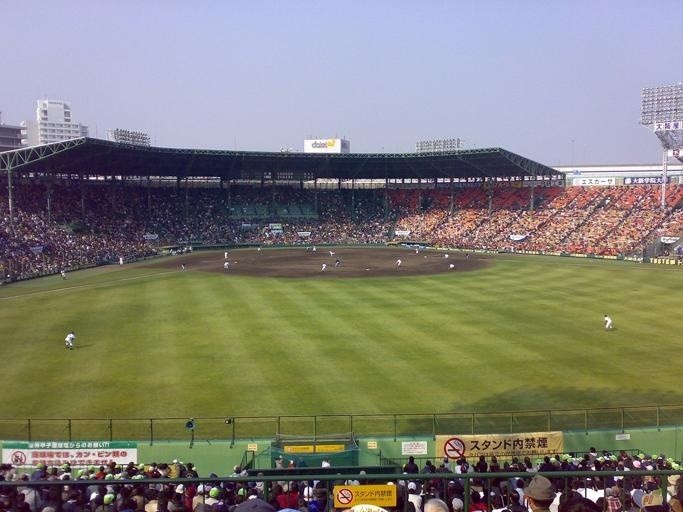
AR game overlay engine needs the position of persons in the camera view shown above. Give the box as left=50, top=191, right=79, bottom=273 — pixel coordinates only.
left=424, top=185, right=682, bottom=264
left=334, top=259, right=341, bottom=268
left=394, top=258, right=401, bottom=272
left=224, top=251, right=228, bottom=262
left=2, top=447, right=683, bottom=512
left=602, top=314, right=612, bottom=330
left=0, top=170, right=193, bottom=284
left=328, top=250, right=335, bottom=256
left=63, top=330, right=75, bottom=350
left=223, top=261, right=230, bottom=272
left=192, top=185, right=423, bottom=255
left=447, top=264, right=455, bottom=272
left=320, top=263, right=327, bottom=272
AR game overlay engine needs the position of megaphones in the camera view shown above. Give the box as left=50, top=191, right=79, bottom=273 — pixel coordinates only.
left=186, top=421, right=195, bottom=430
